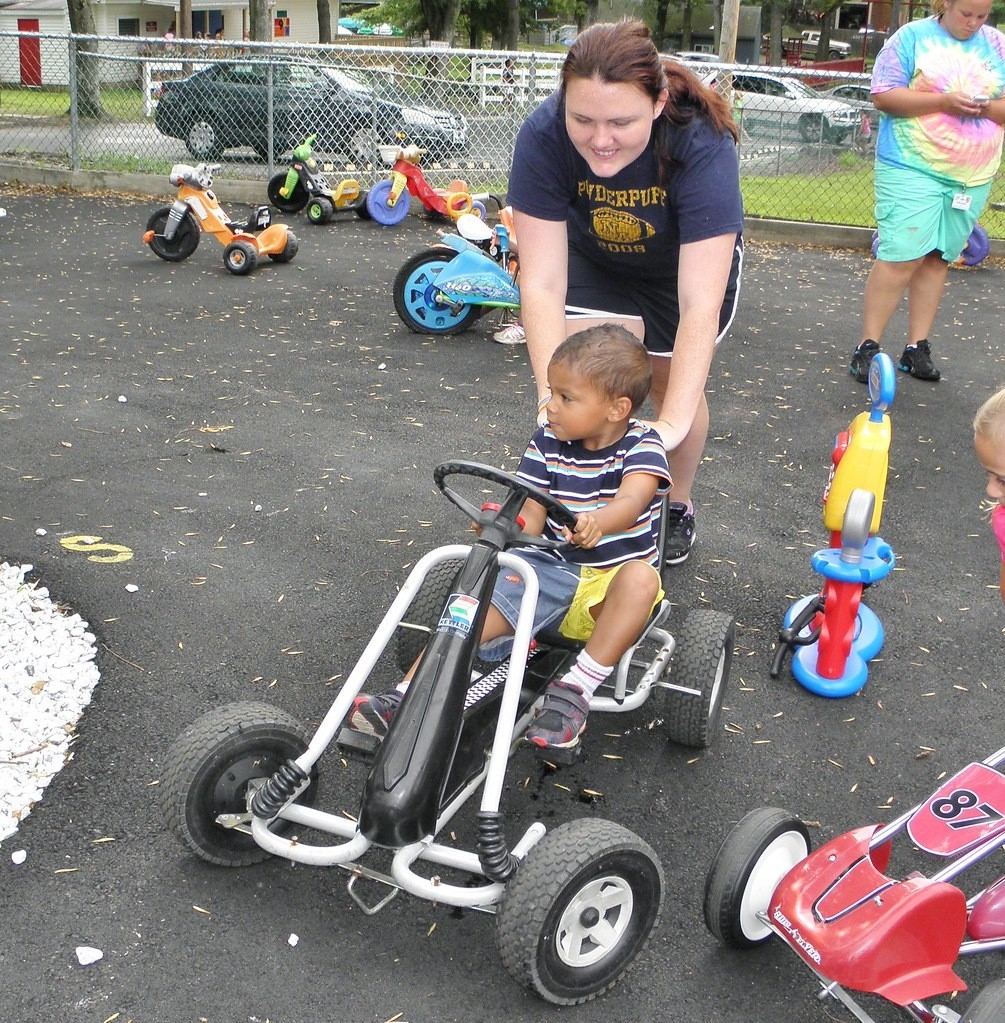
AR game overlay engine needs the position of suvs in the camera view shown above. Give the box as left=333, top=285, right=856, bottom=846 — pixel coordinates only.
left=701, top=70, right=864, bottom=146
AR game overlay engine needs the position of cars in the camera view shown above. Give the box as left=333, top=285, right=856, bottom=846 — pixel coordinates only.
left=154, top=52, right=472, bottom=165
left=550, top=25, right=579, bottom=46
left=659, top=49, right=881, bottom=126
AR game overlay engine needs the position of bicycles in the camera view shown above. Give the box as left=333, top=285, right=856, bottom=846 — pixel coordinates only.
left=393, top=191, right=524, bottom=335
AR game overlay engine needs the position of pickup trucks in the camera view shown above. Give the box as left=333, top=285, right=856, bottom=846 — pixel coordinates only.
left=762, top=27, right=853, bottom=60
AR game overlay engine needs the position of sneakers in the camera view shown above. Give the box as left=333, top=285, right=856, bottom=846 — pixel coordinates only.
left=525, top=680, right=590, bottom=747
left=850, top=339, right=881, bottom=383
left=898, top=339, right=940, bottom=382
left=349, top=687, right=406, bottom=738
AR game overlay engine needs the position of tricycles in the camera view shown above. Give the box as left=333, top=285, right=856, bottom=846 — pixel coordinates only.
left=367, top=143, right=487, bottom=225
left=142, top=161, right=298, bottom=276
left=867, top=217, right=991, bottom=268
left=268, top=133, right=375, bottom=224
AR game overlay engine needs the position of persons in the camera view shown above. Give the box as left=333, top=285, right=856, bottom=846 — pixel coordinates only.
left=502, top=57, right=519, bottom=116
left=848, top=1, right=1004, bottom=385
left=970, top=385, right=1005, bottom=611
left=342, top=323, right=679, bottom=752
left=164, top=24, right=255, bottom=60
left=503, top=17, right=747, bottom=571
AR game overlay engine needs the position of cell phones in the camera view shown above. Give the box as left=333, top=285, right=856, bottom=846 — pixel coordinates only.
left=971, top=95, right=989, bottom=102
